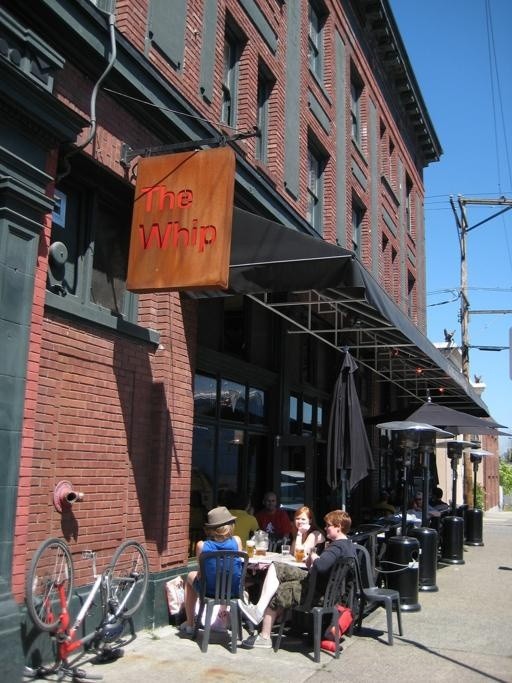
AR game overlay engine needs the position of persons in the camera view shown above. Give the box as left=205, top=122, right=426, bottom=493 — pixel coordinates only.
left=236, top=509, right=356, bottom=648
left=430, top=488, right=449, bottom=511
left=412, top=491, right=441, bottom=518
left=178, top=505, right=242, bottom=638
left=374, top=494, right=397, bottom=514
left=290, top=506, right=328, bottom=562
left=254, top=490, right=292, bottom=537
left=228, top=495, right=261, bottom=546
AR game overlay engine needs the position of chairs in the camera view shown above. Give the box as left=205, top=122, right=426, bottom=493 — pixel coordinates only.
left=274, top=556, right=354, bottom=663
left=193, top=551, right=249, bottom=653
left=266, top=502, right=471, bottom=558
left=350, top=544, right=403, bottom=646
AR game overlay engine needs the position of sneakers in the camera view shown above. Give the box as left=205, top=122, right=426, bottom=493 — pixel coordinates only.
left=238, top=599, right=263, bottom=626
left=180, top=624, right=195, bottom=638
left=242, top=633, right=272, bottom=649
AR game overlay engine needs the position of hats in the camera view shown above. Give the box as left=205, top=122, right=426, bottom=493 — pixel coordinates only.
left=204, top=506, right=238, bottom=526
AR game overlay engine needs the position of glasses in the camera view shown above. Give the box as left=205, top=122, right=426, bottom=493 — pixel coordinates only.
left=326, top=523, right=334, bottom=527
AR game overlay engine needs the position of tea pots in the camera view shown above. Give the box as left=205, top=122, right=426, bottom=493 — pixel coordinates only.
left=254, top=530, right=268, bottom=557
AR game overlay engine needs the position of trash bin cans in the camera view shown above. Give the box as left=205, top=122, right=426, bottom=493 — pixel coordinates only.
left=439, top=516, right=465, bottom=564
left=465, top=509, right=484, bottom=546
left=385, top=527, right=438, bottom=612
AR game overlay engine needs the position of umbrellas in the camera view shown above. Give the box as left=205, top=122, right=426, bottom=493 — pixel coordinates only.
left=328, top=345, right=378, bottom=510
left=438, top=425, right=512, bottom=438
left=361, top=400, right=509, bottom=429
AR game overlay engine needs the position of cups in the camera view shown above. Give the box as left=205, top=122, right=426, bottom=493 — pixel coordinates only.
left=282, top=545, right=290, bottom=559
left=294, top=548, right=305, bottom=563
left=245, top=541, right=254, bottom=558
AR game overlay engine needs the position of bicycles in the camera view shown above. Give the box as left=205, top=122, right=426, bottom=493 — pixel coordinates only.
left=22, top=537, right=150, bottom=680
